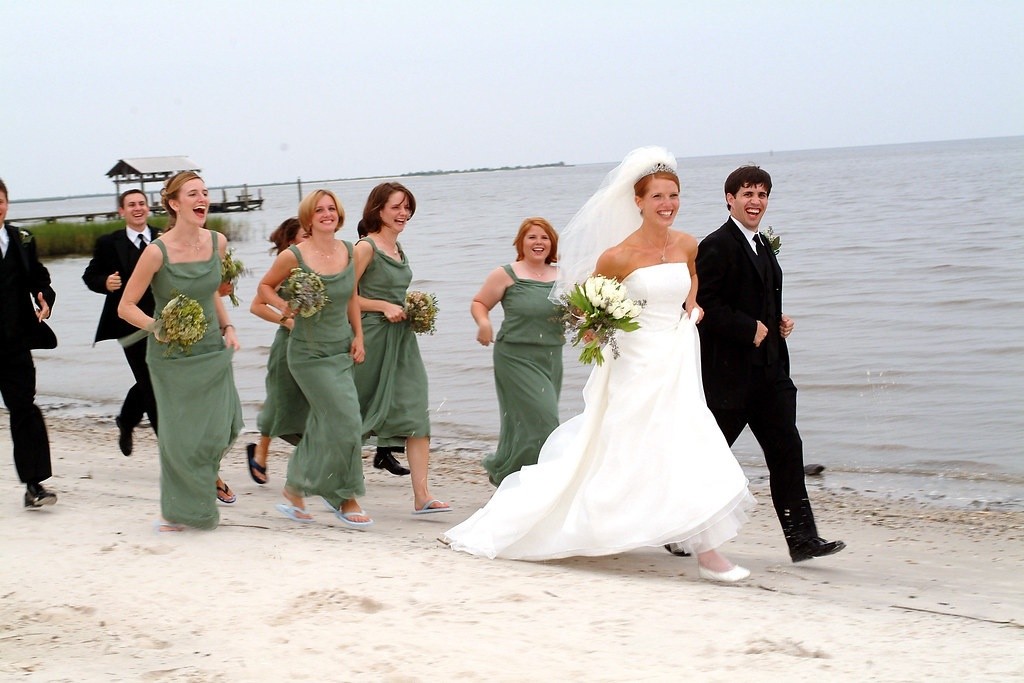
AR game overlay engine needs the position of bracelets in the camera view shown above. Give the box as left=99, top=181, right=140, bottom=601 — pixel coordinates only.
left=224, top=325, right=234, bottom=330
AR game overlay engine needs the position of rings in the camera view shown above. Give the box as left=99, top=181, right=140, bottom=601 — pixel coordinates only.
left=788, top=331, right=790, bottom=334
left=584, top=334, right=587, bottom=338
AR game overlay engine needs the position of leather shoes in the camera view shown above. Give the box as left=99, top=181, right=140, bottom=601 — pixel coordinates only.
left=791, top=536, right=847, bottom=562
left=664, top=541, right=692, bottom=557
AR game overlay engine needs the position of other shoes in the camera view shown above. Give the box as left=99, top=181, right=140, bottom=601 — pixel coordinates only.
left=697, top=561, right=752, bottom=584
left=373, top=451, right=412, bottom=477
left=115, top=415, right=133, bottom=457
left=23, top=484, right=58, bottom=511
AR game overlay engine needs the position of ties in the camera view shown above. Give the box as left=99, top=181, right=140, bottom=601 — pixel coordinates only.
left=137, top=234, right=147, bottom=249
left=750, top=233, right=771, bottom=284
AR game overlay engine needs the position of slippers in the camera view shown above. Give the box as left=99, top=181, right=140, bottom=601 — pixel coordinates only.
left=334, top=508, right=374, bottom=527
left=246, top=444, right=267, bottom=484
left=272, top=498, right=315, bottom=524
left=157, top=515, right=182, bottom=532
left=215, top=478, right=236, bottom=504
left=412, top=499, right=454, bottom=515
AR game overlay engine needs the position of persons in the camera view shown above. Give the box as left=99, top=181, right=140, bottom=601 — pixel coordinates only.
left=472, top=216, right=579, bottom=489
left=246, top=182, right=452, bottom=528
left=0, top=178, right=57, bottom=507
left=442, top=145, right=758, bottom=582
left=116, top=170, right=244, bottom=531
left=661, top=165, right=845, bottom=564
left=82, top=189, right=165, bottom=455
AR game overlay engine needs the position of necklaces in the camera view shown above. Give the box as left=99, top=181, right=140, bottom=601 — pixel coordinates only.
left=183, top=236, right=200, bottom=250
left=640, top=227, right=669, bottom=261
left=523, top=259, right=549, bottom=278
left=311, top=244, right=338, bottom=259
left=395, top=251, right=396, bottom=254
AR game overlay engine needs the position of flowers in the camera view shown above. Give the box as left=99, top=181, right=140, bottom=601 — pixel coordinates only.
left=279, top=266, right=333, bottom=324
left=19, top=230, right=35, bottom=247
left=157, top=289, right=211, bottom=357
left=382, top=290, right=439, bottom=336
left=763, top=226, right=781, bottom=255
left=561, top=273, right=643, bottom=367
left=222, top=247, right=244, bottom=306
